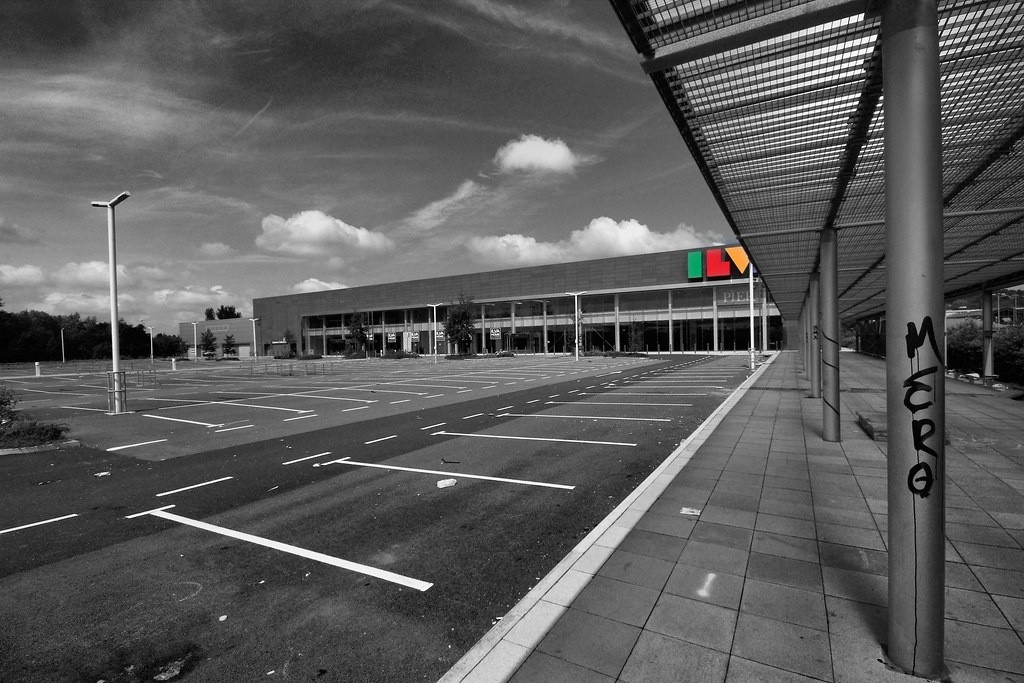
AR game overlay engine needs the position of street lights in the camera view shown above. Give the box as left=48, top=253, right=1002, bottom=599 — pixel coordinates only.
left=148, top=326, right=155, bottom=363
left=91, top=191, right=132, bottom=414
left=191, top=322, right=200, bottom=362
left=248, top=317, right=259, bottom=365
left=564, top=291, right=588, bottom=361
left=427, top=303, right=442, bottom=363
